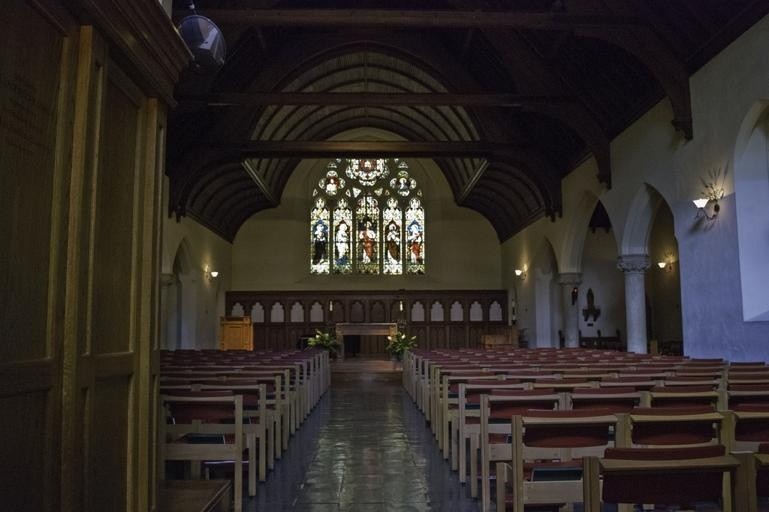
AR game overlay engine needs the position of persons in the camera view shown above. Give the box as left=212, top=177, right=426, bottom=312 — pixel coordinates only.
left=313, top=220, right=422, bottom=265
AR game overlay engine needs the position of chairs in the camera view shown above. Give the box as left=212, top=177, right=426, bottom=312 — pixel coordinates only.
left=394, top=345, right=769, bottom=511
left=160, top=349, right=330, bottom=507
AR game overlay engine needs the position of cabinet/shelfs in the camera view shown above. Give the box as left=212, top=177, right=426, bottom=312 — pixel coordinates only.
left=1, top=2, right=206, bottom=512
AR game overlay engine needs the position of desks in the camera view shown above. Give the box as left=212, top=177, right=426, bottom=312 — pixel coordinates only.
left=335, top=323, right=398, bottom=356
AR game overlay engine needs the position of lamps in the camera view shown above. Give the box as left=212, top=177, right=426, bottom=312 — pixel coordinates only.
left=693, top=194, right=722, bottom=222
left=514, top=269, right=528, bottom=281
